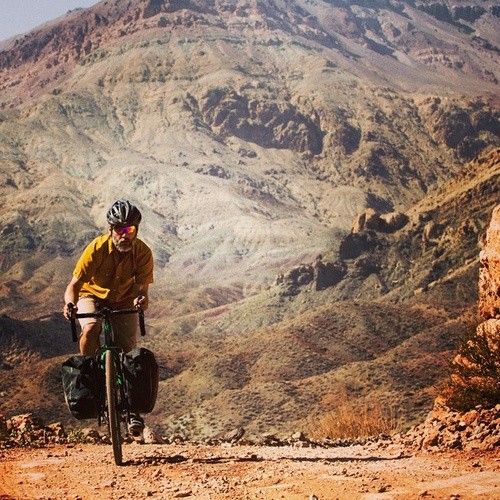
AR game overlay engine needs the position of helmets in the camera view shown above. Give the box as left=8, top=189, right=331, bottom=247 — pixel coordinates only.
left=106, top=198, right=142, bottom=225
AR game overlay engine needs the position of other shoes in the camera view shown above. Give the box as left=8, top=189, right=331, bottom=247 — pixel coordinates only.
left=128, top=410, right=145, bottom=437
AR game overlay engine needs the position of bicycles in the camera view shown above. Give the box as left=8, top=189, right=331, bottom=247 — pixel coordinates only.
left=64, top=296, right=146, bottom=465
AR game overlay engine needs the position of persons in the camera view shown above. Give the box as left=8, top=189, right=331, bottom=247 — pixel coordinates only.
left=62, top=199, right=155, bottom=438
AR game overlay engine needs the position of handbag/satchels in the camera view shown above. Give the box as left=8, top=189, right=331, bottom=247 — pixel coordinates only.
left=61, top=355, right=104, bottom=420
left=122, top=347, right=158, bottom=413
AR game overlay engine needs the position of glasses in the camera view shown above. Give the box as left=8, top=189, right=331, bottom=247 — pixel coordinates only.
left=111, top=225, right=136, bottom=235
